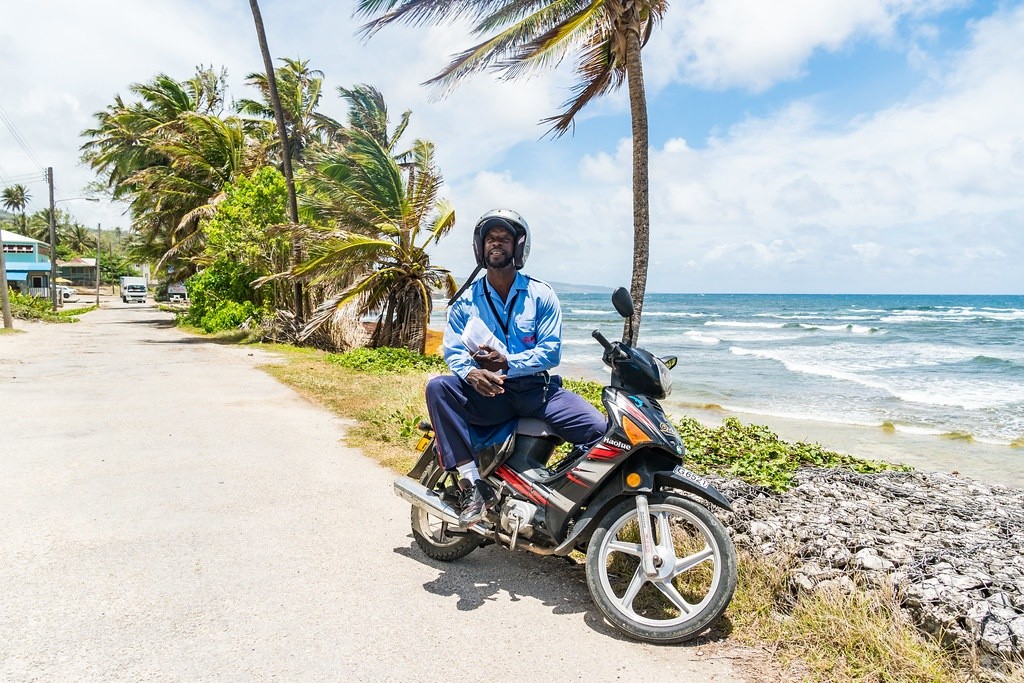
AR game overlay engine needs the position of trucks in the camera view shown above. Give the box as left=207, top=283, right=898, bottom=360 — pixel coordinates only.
left=121, top=278, right=147, bottom=303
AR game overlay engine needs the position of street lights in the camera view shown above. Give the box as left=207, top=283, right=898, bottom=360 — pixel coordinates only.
left=49, top=198, right=100, bottom=312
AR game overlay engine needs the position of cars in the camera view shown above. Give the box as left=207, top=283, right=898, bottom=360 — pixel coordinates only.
left=56, top=285, right=77, bottom=299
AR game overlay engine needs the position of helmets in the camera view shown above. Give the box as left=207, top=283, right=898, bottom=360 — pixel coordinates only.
left=472, top=207, right=531, bottom=270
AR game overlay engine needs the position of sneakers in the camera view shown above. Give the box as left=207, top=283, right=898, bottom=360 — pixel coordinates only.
left=454, top=478, right=502, bottom=528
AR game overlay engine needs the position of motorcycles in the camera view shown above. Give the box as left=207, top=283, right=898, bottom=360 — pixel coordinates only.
left=392, top=281, right=739, bottom=646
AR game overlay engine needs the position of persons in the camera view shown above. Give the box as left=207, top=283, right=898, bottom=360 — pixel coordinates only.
left=424, top=207, right=608, bottom=530
